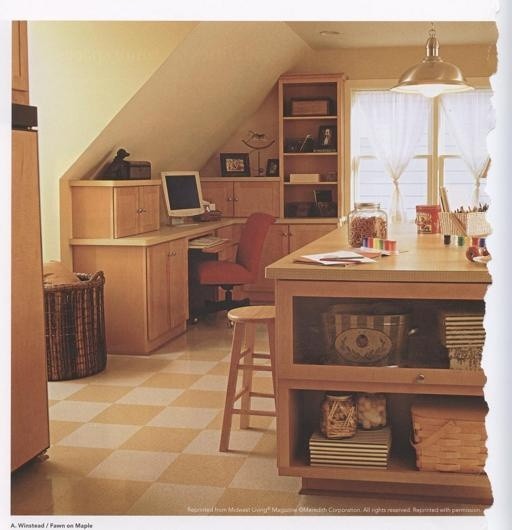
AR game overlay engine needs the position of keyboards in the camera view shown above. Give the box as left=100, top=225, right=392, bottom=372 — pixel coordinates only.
left=190, top=235, right=224, bottom=249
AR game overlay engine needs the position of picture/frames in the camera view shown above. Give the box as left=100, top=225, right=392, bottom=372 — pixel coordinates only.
left=219, top=152, right=251, bottom=176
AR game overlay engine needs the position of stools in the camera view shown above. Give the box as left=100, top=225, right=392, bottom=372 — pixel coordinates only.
left=218, top=307, right=275, bottom=453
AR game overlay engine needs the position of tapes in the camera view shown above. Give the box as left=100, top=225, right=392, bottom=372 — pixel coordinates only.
left=465, top=247, right=480, bottom=263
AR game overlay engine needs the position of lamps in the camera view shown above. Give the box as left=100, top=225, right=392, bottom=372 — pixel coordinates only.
left=386, top=22, right=474, bottom=97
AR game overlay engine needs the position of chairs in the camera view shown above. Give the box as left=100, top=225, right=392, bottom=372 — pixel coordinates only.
left=195, top=213, right=277, bottom=329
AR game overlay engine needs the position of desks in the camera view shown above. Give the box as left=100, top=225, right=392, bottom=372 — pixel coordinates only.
left=69, top=217, right=233, bottom=354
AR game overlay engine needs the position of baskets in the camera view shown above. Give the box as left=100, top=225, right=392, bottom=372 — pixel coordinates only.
left=43, top=270, right=108, bottom=381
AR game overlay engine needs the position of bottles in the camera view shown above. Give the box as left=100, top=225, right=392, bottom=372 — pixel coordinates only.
left=347, top=202, right=390, bottom=249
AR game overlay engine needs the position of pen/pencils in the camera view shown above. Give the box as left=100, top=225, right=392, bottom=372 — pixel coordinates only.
left=439, top=187, right=489, bottom=213
left=320, top=257, right=363, bottom=263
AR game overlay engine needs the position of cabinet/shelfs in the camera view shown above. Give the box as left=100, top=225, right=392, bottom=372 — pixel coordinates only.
left=264, top=222, right=493, bottom=503
left=277, top=71, right=348, bottom=225
left=235, top=223, right=340, bottom=304
left=68, top=179, right=279, bottom=238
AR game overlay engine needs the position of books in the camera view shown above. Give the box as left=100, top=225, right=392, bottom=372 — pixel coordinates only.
left=301, top=250, right=377, bottom=265
left=293, top=248, right=382, bottom=268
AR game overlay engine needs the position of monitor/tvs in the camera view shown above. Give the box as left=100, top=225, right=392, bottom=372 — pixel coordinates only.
left=160, top=171, right=205, bottom=226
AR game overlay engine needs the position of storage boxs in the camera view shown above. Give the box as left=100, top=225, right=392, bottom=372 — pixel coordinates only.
left=307, top=419, right=392, bottom=469
left=439, top=307, right=485, bottom=371
